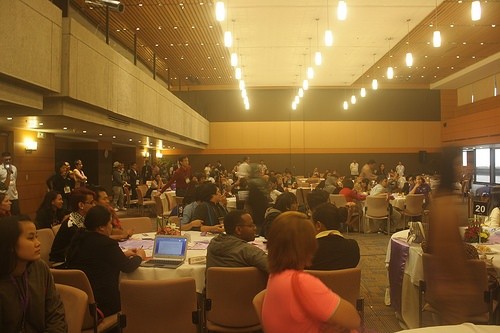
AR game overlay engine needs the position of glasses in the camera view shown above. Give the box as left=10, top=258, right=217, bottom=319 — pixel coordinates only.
left=235, top=224, right=257, bottom=228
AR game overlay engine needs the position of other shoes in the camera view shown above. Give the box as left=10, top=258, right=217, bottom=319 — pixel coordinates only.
left=119, top=207, right=127, bottom=210
left=114, top=207, right=119, bottom=212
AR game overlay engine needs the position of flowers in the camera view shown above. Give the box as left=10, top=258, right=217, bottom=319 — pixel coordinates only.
left=465, top=227, right=490, bottom=244
left=157, top=217, right=183, bottom=239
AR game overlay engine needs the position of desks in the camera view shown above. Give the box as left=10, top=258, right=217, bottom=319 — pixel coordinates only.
left=389, top=237, right=500, bottom=319
left=110, top=232, right=268, bottom=291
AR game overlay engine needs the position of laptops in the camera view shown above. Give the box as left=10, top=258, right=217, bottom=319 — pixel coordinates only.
left=139, top=235, right=188, bottom=270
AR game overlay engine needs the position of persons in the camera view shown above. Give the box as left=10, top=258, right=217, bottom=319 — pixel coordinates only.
left=305, top=203, right=364, bottom=270
left=262, top=193, right=298, bottom=238
left=488, top=206, right=500, bottom=228
left=202, top=210, right=270, bottom=319
left=253, top=209, right=361, bottom=333
left=111, top=154, right=302, bottom=212
left=91, top=185, right=135, bottom=242
left=0, top=191, right=11, bottom=216
left=46, top=159, right=87, bottom=220
left=37, top=190, right=64, bottom=230
left=0, top=214, right=67, bottom=333
left=48, top=189, right=95, bottom=270
left=181, top=181, right=226, bottom=233
left=308, top=158, right=431, bottom=235
left=65, top=205, right=146, bottom=333
left=0, top=150, right=19, bottom=216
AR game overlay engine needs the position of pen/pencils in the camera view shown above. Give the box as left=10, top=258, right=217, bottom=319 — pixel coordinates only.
left=140, top=246, right=144, bottom=250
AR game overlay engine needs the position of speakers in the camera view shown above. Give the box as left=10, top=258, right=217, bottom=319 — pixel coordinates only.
left=419, top=150, right=427, bottom=163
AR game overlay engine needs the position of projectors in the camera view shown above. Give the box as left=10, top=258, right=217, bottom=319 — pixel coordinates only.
left=85, top=0, right=125, bottom=14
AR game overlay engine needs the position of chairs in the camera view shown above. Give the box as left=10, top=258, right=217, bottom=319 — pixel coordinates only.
left=125, top=186, right=175, bottom=218
left=50, top=266, right=362, bottom=333
left=421, top=253, right=495, bottom=326
left=298, top=188, right=429, bottom=235
